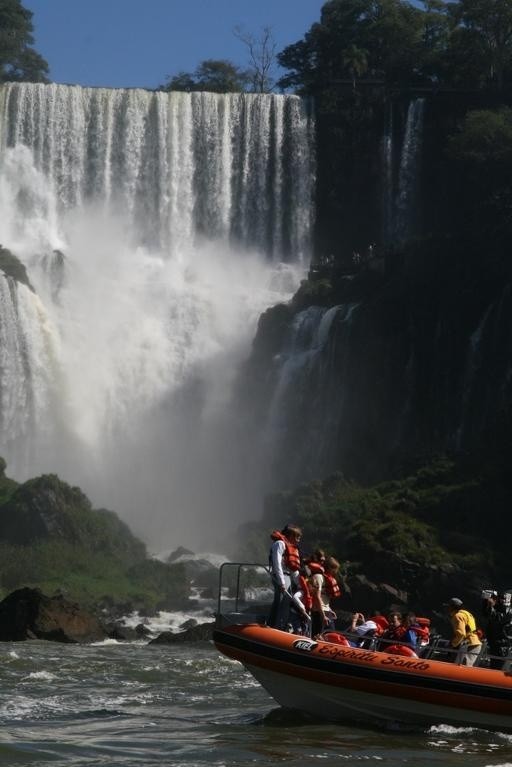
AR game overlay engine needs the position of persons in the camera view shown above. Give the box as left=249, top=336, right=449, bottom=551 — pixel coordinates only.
left=293, top=548, right=512, bottom=670
left=269, top=525, right=303, bottom=632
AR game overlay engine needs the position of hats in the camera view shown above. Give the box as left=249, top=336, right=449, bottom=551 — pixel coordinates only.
left=442, top=598, right=463, bottom=608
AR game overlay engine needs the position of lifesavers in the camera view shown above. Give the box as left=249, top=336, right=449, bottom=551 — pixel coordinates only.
left=383, top=645, right=418, bottom=658
left=322, top=633, right=350, bottom=647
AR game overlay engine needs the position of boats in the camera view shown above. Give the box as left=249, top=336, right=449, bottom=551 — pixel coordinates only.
left=212, top=562, right=512, bottom=735
